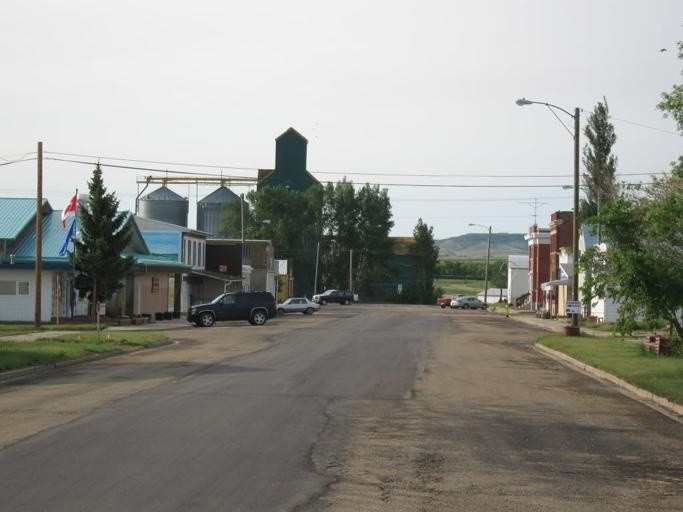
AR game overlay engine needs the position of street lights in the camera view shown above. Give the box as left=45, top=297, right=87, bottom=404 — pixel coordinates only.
left=515, top=97, right=582, bottom=335
left=559, top=182, right=602, bottom=247
left=468, top=223, right=492, bottom=306
left=240, top=218, right=271, bottom=245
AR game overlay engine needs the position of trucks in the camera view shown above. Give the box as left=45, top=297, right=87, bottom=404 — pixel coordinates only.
left=435, top=292, right=465, bottom=308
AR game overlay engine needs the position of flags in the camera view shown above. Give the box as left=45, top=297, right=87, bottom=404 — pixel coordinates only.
left=58, top=218, right=76, bottom=257
left=60, top=193, right=77, bottom=231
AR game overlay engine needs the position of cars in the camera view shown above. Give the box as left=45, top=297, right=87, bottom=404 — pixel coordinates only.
left=450, top=296, right=489, bottom=310
left=277, top=298, right=320, bottom=314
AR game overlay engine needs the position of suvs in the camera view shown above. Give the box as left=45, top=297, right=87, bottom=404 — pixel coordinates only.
left=187, top=290, right=277, bottom=327
left=312, top=289, right=353, bottom=305
